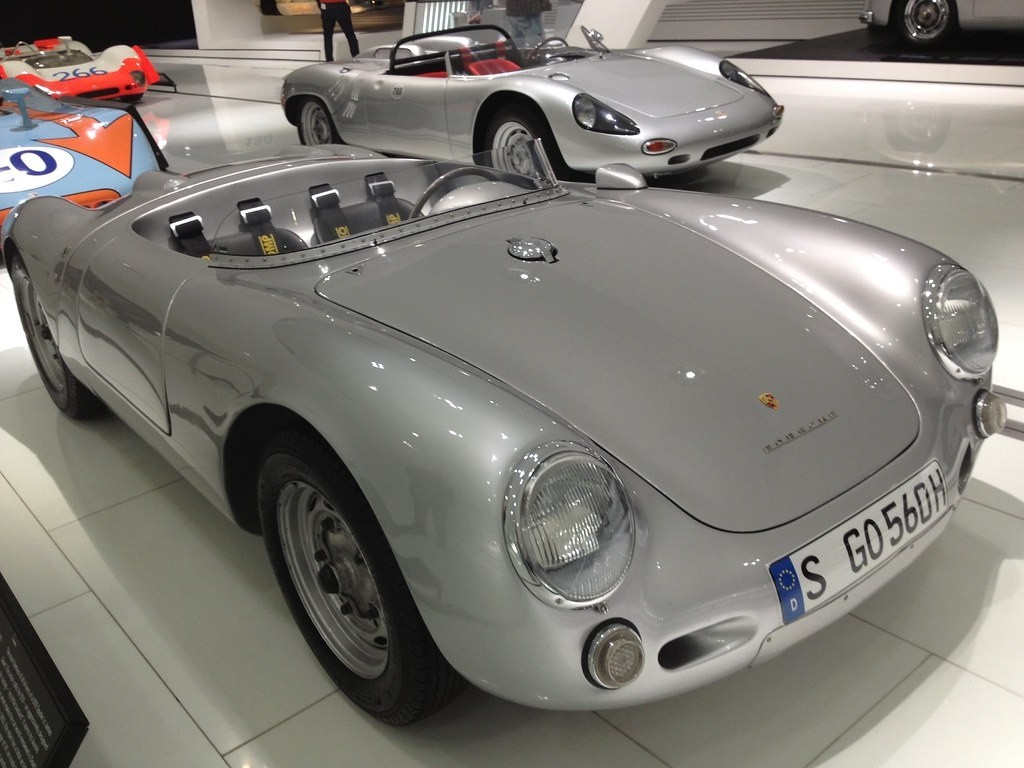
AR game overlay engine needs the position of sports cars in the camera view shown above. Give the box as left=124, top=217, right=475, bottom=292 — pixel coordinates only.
left=1, top=78, right=170, bottom=245
left=0, top=35, right=160, bottom=104
left=280, top=24, right=786, bottom=183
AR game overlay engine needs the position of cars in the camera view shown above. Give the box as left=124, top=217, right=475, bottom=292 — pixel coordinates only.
left=1, top=141, right=1011, bottom=725
left=857, top=0, right=1024, bottom=53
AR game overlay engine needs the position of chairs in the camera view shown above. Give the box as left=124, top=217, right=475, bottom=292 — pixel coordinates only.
left=470, top=56, right=521, bottom=74
left=183, top=227, right=310, bottom=258
left=314, top=199, right=423, bottom=245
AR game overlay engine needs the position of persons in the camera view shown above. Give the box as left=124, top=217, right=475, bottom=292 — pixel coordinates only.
left=506, top=0, right=552, bottom=48
left=317, top=0, right=360, bottom=62
